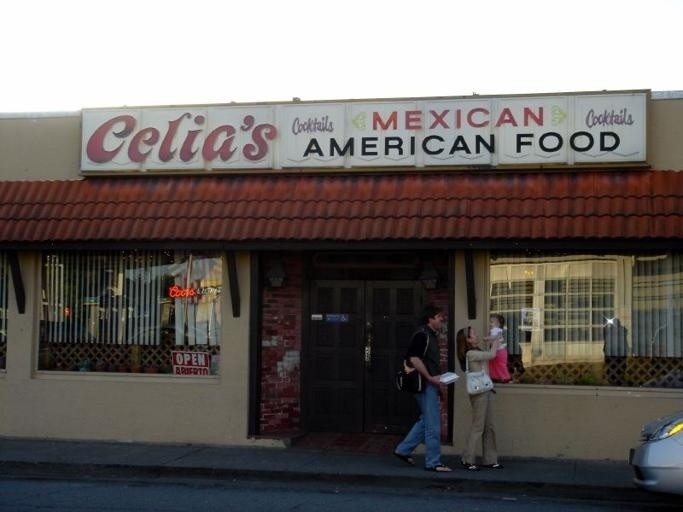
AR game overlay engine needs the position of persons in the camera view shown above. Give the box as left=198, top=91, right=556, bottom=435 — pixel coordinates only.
left=394, top=306, right=454, bottom=472
left=482, top=313, right=510, bottom=383
left=457, top=326, right=503, bottom=470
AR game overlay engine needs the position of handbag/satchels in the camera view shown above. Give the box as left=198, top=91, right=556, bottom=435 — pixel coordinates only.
left=396, top=360, right=425, bottom=394
left=465, top=351, right=494, bottom=395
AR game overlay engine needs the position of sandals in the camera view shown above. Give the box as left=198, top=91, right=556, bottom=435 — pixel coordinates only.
left=393, top=449, right=415, bottom=465
left=424, top=464, right=453, bottom=472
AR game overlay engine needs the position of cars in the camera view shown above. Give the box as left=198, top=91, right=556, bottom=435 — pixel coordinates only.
left=629, top=411, right=683, bottom=496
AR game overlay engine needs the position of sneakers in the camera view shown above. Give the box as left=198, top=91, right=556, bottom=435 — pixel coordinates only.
left=464, top=463, right=480, bottom=471
left=483, top=463, right=503, bottom=469
